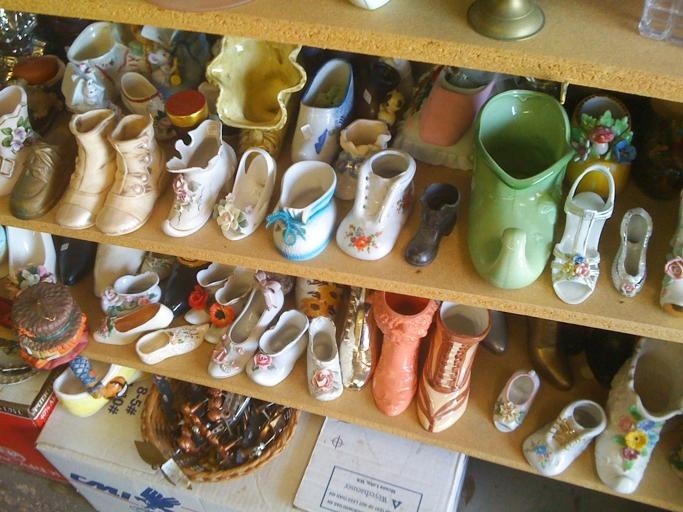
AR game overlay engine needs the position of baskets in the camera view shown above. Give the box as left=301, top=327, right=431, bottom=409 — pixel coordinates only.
left=141, top=375, right=301, bottom=483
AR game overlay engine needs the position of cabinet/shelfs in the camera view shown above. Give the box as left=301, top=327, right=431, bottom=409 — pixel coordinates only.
left=0, top=2, right=683, bottom=512
